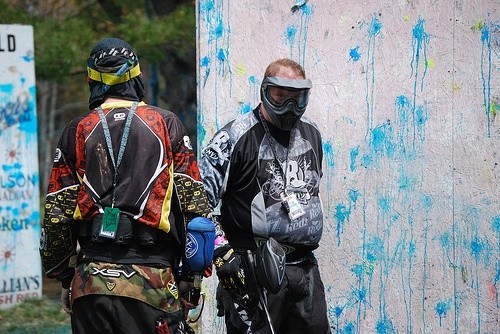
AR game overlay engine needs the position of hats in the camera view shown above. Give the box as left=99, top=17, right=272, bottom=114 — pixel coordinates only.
left=86, top=37, right=145, bottom=110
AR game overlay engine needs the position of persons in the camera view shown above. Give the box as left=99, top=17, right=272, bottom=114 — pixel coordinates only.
left=199, top=59, right=332, bottom=334
left=39, top=38, right=211, bottom=334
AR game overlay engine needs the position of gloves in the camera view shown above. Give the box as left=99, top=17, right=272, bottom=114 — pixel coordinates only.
left=213, top=244, right=247, bottom=292
